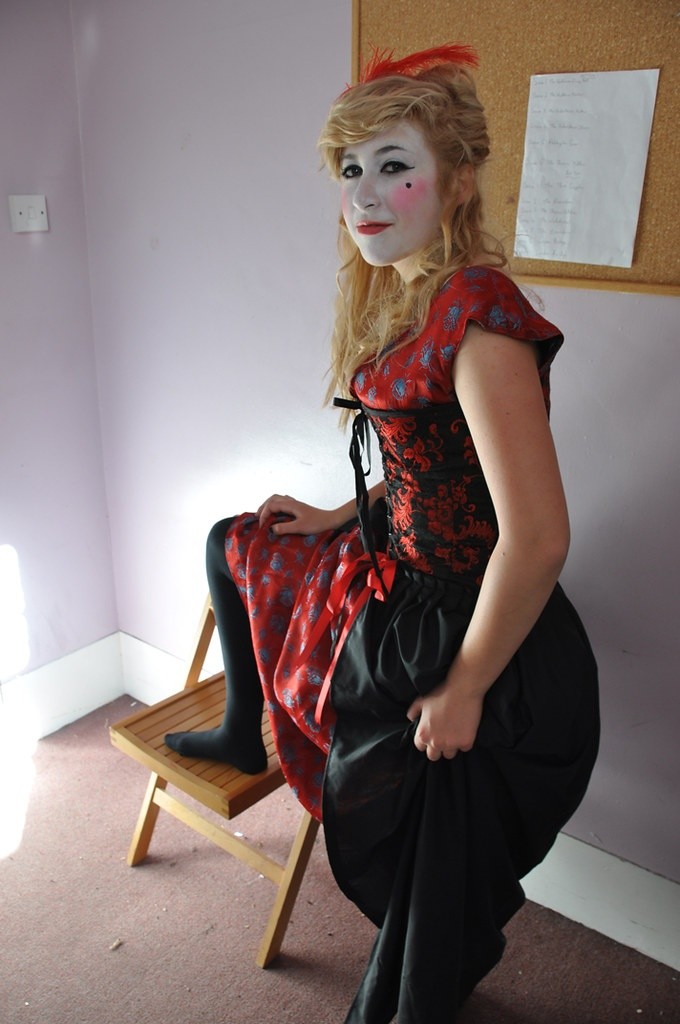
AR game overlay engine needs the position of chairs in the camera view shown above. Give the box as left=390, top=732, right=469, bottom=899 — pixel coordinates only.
left=104, top=585, right=322, bottom=973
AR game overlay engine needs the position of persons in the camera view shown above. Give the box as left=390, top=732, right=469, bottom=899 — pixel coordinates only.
left=164, top=42, right=601, bottom=1024
